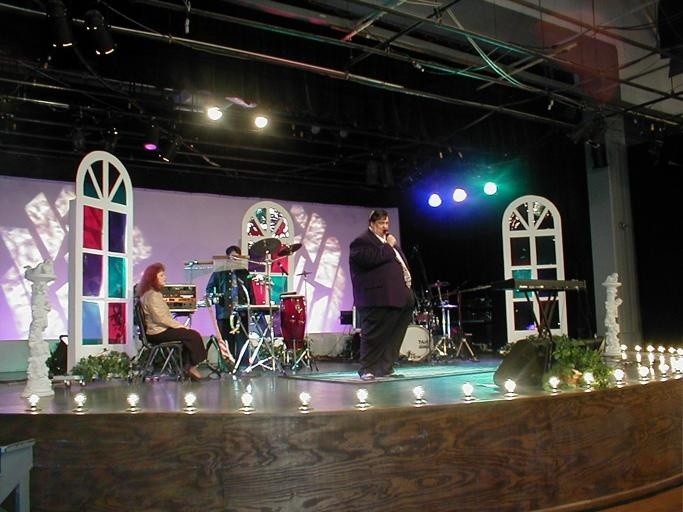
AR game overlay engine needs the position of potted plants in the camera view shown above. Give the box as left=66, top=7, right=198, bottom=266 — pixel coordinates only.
left=530, top=332, right=612, bottom=393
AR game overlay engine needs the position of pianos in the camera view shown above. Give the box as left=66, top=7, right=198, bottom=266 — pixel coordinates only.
left=492, top=278, right=586, bottom=290
left=232, top=303, right=280, bottom=313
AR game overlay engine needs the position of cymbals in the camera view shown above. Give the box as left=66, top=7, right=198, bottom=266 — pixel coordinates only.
left=251, top=238, right=281, bottom=255
left=278, top=243, right=303, bottom=256
left=297, top=272, right=313, bottom=276
left=415, top=314, right=436, bottom=323
left=438, top=305, right=459, bottom=308
left=213, top=263, right=247, bottom=272
left=213, top=255, right=246, bottom=259
left=428, top=282, right=451, bottom=287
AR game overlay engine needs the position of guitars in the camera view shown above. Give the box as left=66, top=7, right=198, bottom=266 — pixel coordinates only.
left=203, top=295, right=237, bottom=372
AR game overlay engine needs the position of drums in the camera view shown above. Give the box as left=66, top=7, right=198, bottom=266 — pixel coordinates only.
left=280, top=295, right=307, bottom=349
left=450, top=327, right=461, bottom=339
left=399, top=325, right=434, bottom=362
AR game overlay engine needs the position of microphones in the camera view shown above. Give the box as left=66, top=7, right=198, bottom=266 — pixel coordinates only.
left=383, top=229, right=400, bottom=249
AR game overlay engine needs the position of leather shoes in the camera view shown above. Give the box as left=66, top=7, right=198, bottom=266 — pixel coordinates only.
left=361, top=372, right=375, bottom=380
left=183, top=370, right=211, bottom=382
left=388, top=371, right=404, bottom=378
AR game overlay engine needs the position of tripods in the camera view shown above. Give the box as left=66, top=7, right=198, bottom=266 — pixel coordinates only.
left=429, top=286, right=457, bottom=357
left=228, top=263, right=286, bottom=375
left=291, top=278, right=320, bottom=373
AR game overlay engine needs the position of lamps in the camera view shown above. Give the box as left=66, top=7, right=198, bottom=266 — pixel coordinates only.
left=636, top=352, right=641, bottom=360
left=126, top=393, right=143, bottom=411
left=677, top=348, right=683, bottom=354
left=648, top=353, right=655, bottom=362
left=678, top=357, right=683, bottom=362
left=145, top=124, right=160, bottom=149
left=36, top=31, right=77, bottom=70
left=660, top=354, right=666, bottom=363
left=659, top=363, right=669, bottom=378
left=84, top=19, right=118, bottom=55
left=297, top=392, right=315, bottom=414
left=635, top=345, right=641, bottom=352
left=658, top=346, right=666, bottom=353
left=410, top=386, right=427, bottom=407
left=621, top=343, right=627, bottom=352
left=353, top=387, right=371, bottom=410
left=28, top=393, right=41, bottom=412
left=668, top=347, right=675, bottom=353
left=457, top=381, right=476, bottom=401
left=670, top=355, right=676, bottom=361
left=502, top=379, right=521, bottom=399
left=638, top=366, right=650, bottom=382
left=237, top=391, right=256, bottom=413
left=548, top=377, right=562, bottom=395
left=621, top=352, right=628, bottom=360
left=613, top=369, right=627, bottom=385
left=180, top=392, right=201, bottom=414
left=672, top=363, right=683, bottom=374
left=156, top=138, right=177, bottom=163
left=647, top=345, right=655, bottom=353
left=581, top=373, right=597, bottom=392
left=72, top=393, right=89, bottom=412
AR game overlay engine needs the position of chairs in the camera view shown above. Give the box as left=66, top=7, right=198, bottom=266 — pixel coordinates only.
left=130, top=302, right=182, bottom=381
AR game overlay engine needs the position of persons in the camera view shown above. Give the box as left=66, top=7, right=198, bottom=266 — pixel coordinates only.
left=348, top=209, right=415, bottom=380
left=138, top=263, right=206, bottom=382
left=206, top=246, right=256, bottom=371
